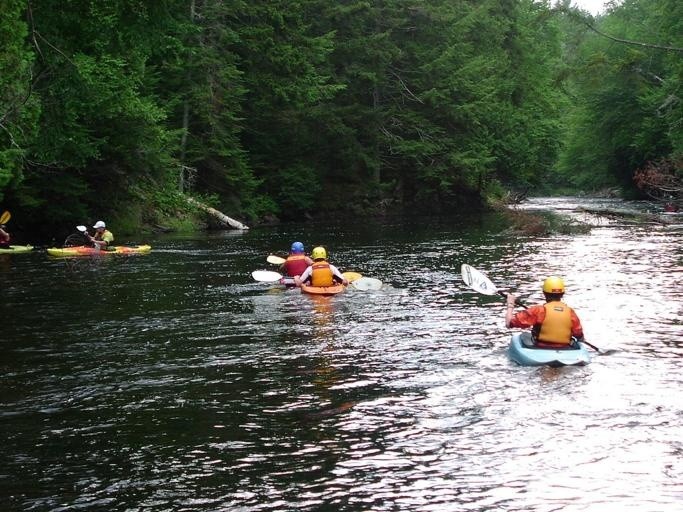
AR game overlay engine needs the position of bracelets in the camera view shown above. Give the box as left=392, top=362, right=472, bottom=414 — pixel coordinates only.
left=507, top=302, right=513, bottom=308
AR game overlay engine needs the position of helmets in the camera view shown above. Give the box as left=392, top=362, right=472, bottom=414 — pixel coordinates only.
left=543, top=276, right=564, bottom=294
left=291, top=242, right=304, bottom=255
left=312, top=247, right=326, bottom=260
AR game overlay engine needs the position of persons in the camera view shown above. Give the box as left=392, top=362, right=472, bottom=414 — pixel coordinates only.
left=294, top=247, right=344, bottom=286
left=83, top=221, right=114, bottom=250
left=504, top=276, right=585, bottom=351
left=277, top=241, right=315, bottom=278
left=0, top=225, right=10, bottom=246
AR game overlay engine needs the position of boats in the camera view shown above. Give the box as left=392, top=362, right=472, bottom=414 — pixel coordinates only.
left=280, top=275, right=310, bottom=289
left=297, top=270, right=362, bottom=296
left=0, top=245, right=39, bottom=255
left=46, top=243, right=155, bottom=256
left=504, top=323, right=591, bottom=370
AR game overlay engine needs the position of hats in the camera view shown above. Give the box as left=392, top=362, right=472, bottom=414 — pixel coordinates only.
left=93, top=221, right=106, bottom=228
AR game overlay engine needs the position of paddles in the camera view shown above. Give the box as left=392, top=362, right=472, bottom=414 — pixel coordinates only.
left=460, top=264, right=605, bottom=355
left=76, top=225, right=101, bottom=254
left=0, top=210, right=11, bottom=224
left=252, top=255, right=382, bottom=291
